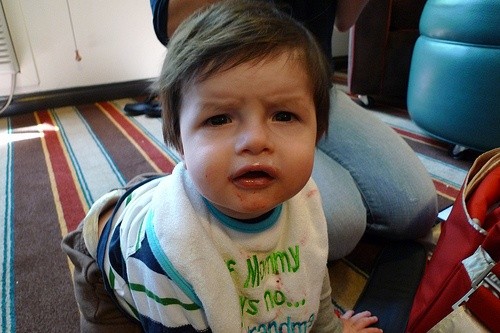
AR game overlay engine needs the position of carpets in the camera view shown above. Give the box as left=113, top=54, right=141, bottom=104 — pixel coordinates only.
left=1, top=67, right=480, bottom=333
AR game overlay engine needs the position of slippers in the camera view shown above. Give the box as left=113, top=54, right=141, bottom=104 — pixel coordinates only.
left=124, top=93, right=163, bottom=116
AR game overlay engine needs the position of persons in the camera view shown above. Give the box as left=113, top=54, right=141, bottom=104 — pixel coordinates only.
left=149, top=0, right=438, bottom=262
left=61, top=0, right=383, bottom=333
